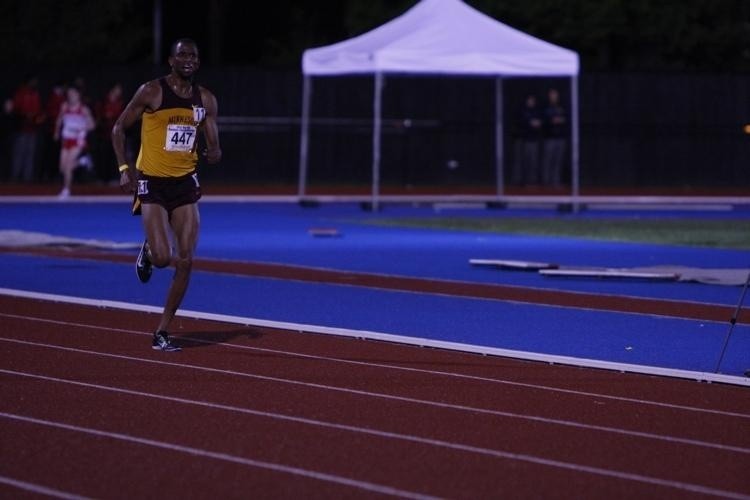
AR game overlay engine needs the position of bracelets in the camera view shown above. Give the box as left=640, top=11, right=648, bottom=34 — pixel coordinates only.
left=118, top=164, right=129, bottom=173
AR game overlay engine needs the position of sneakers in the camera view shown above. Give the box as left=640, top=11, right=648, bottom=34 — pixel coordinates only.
left=135, top=236, right=158, bottom=285
left=151, top=330, right=183, bottom=353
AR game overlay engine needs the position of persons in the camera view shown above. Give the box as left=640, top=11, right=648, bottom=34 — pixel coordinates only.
left=540, top=88, right=567, bottom=186
left=93, top=82, right=125, bottom=182
left=510, top=93, right=545, bottom=186
left=2, top=71, right=96, bottom=199
left=110, top=38, right=222, bottom=352
